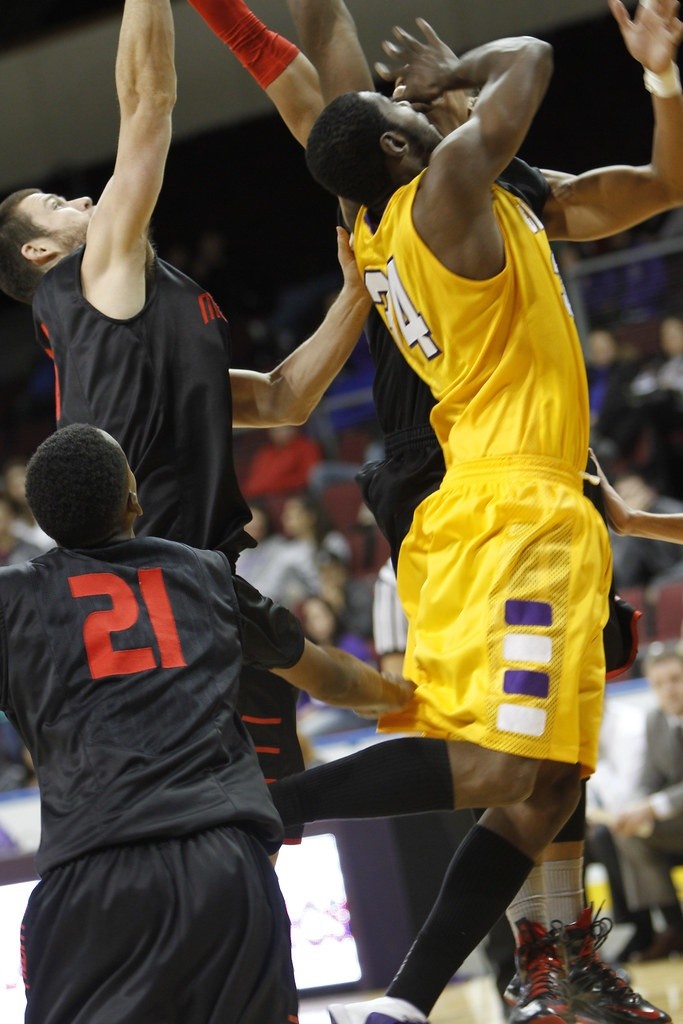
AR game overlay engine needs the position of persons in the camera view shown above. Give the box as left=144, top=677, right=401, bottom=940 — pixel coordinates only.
left=0, top=0, right=683, bottom=1024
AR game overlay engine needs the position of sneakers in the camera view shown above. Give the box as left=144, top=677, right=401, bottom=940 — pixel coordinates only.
left=503, top=917, right=575, bottom=1024
left=548, top=901, right=673, bottom=1024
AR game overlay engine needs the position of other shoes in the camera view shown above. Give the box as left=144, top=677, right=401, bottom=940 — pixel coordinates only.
left=640, top=927, right=677, bottom=960
left=327, top=996, right=428, bottom=1024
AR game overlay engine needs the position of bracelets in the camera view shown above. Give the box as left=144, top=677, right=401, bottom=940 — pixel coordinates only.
left=643, top=60, right=682, bottom=99
